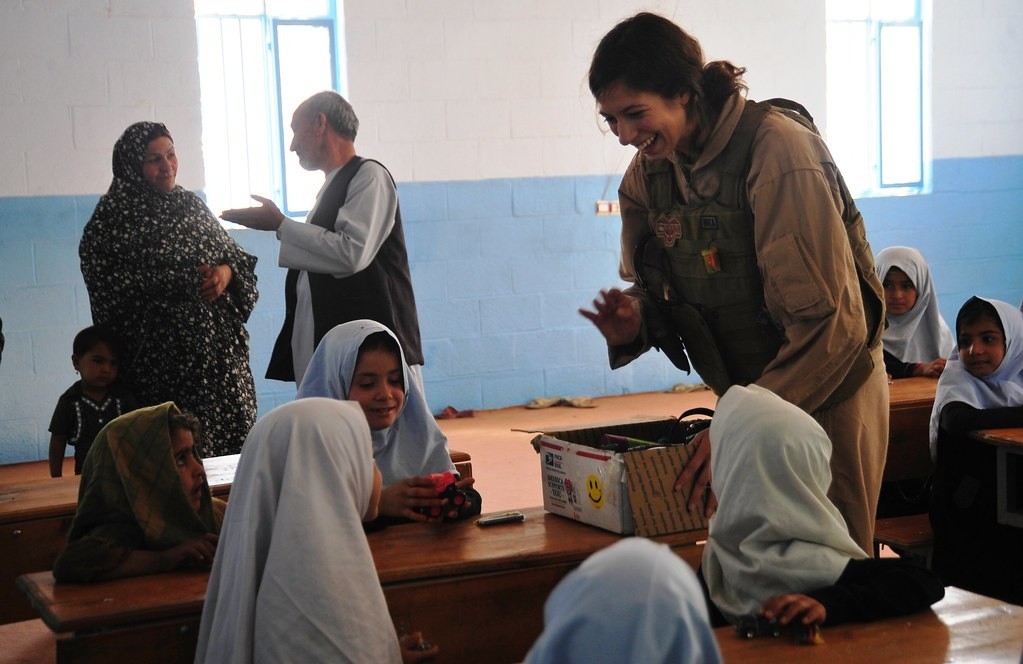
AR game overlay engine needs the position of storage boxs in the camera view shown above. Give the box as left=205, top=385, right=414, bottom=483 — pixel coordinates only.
left=509, top=414, right=720, bottom=536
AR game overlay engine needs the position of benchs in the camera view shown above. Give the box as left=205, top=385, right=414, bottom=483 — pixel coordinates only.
left=871, top=511, right=934, bottom=568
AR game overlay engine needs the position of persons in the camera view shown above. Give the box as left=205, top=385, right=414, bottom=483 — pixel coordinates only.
left=219, top=91, right=424, bottom=390
left=577, top=12, right=890, bottom=558
left=695, top=384, right=945, bottom=628
left=295, top=319, right=475, bottom=522
left=78, top=122, right=258, bottom=456
left=192, top=396, right=440, bottom=664
left=52, top=401, right=226, bottom=585
left=520, top=536, right=723, bottom=664
left=928, top=294, right=1023, bottom=592
left=49, top=326, right=137, bottom=478
left=874, top=246, right=956, bottom=378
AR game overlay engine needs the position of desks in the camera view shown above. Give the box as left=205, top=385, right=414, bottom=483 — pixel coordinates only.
left=706, top=574, right=1023, bottom=664
left=888, top=372, right=942, bottom=433
left=964, top=424, right=1023, bottom=529
left=8, top=504, right=731, bottom=663
left=0, top=451, right=243, bottom=627
